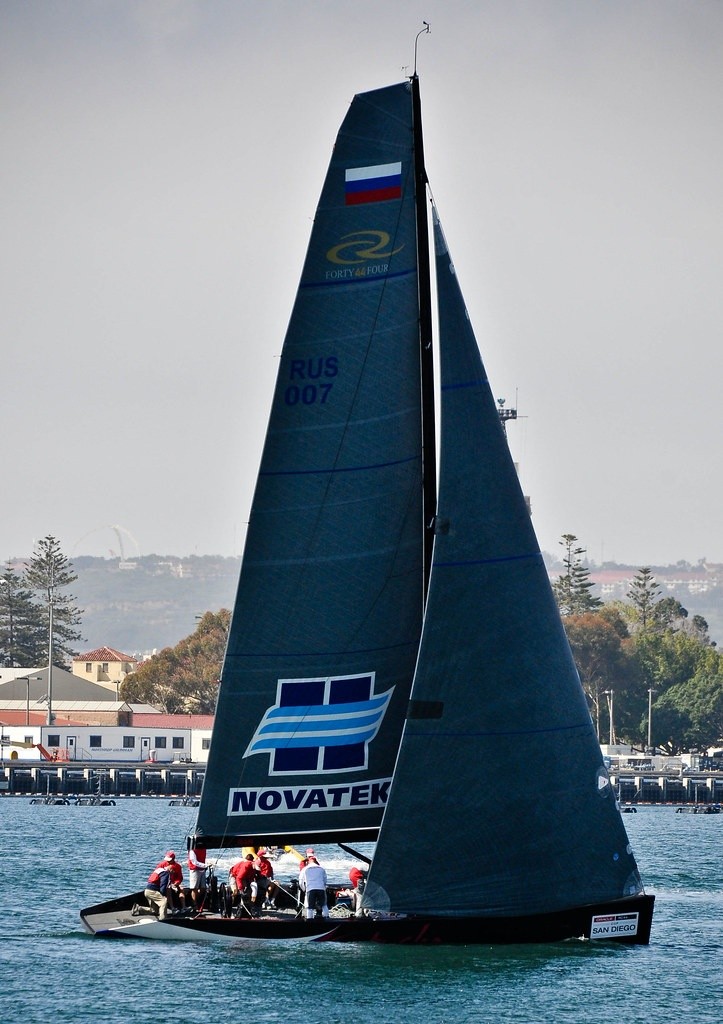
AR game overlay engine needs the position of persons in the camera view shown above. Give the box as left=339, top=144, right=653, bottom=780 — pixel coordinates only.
left=228, top=849, right=280, bottom=918
left=299, top=849, right=329, bottom=919
left=131, top=849, right=212, bottom=921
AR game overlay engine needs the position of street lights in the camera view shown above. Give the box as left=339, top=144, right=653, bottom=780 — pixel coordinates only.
left=15, top=677, right=43, bottom=728
left=112, top=679, right=120, bottom=701
left=603, top=690, right=616, bottom=745
left=46, top=595, right=54, bottom=725
left=647, top=688, right=659, bottom=748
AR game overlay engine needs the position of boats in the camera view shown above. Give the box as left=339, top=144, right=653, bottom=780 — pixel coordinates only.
left=240, top=845, right=306, bottom=865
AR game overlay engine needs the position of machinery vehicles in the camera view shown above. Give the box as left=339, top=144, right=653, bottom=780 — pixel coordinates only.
left=0, top=740, right=70, bottom=764
left=171, top=752, right=197, bottom=765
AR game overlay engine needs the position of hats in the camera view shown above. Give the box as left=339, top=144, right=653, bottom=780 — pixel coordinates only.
left=306, top=848, right=316, bottom=861
left=164, top=851, right=175, bottom=861
left=257, top=849, right=268, bottom=857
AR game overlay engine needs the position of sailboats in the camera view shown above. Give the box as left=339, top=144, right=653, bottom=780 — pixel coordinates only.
left=76, top=20, right=658, bottom=945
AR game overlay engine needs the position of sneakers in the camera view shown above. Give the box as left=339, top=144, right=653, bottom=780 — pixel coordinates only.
left=131, top=903, right=139, bottom=916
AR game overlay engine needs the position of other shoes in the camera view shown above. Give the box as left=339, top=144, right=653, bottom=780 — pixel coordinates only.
left=271, top=903, right=277, bottom=909
left=262, top=903, right=266, bottom=909
